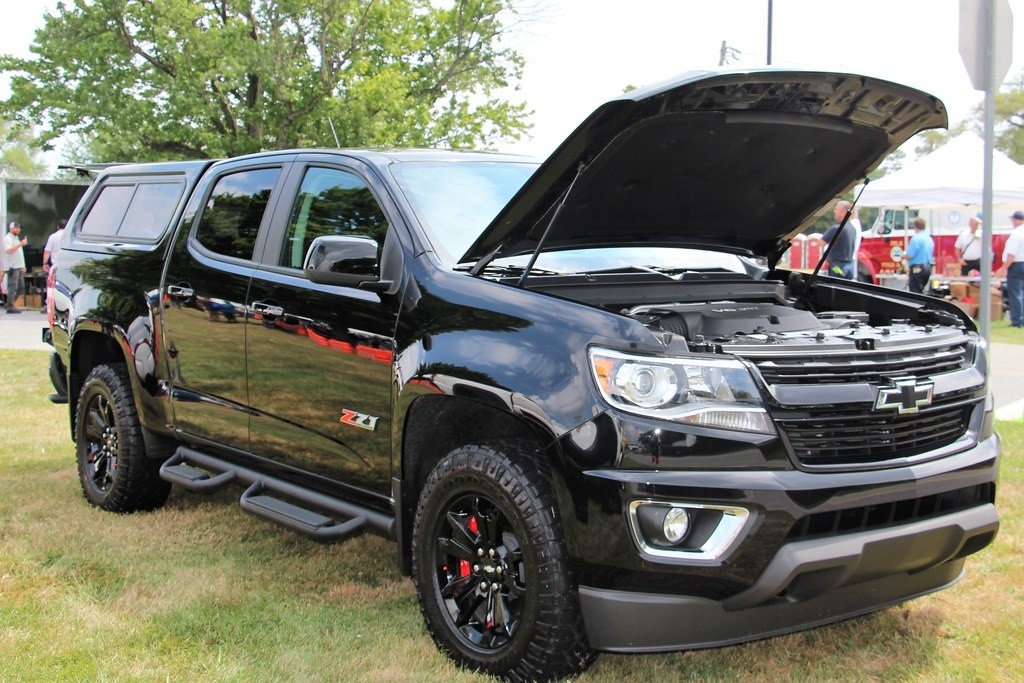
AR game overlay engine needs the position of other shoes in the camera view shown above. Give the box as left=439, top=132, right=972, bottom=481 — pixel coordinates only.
left=6, top=306, right=22, bottom=314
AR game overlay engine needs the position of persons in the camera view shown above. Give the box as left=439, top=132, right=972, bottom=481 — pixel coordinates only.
left=953, top=216, right=982, bottom=276
left=996, top=211, right=1024, bottom=328
left=650, top=433, right=660, bottom=465
left=2, top=221, right=27, bottom=313
left=42, top=219, right=67, bottom=272
left=902, top=217, right=935, bottom=294
left=816, top=200, right=861, bottom=280
left=198, top=296, right=241, bottom=323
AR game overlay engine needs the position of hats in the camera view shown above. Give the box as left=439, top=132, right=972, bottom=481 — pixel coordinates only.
left=1007, top=211, right=1024, bottom=220
left=9, top=221, right=24, bottom=230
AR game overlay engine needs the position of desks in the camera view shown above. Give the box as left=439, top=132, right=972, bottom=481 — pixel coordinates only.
left=873, top=272, right=1009, bottom=296
left=22, top=273, right=48, bottom=307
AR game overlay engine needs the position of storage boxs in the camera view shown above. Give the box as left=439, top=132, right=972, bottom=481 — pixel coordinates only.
left=946, top=281, right=1004, bottom=322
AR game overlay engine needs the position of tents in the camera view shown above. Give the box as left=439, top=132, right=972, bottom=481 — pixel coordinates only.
left=854, top=130, right=1024, bottom=249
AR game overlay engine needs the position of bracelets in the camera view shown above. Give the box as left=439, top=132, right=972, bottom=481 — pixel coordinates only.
left=42, top=263, right=48, bottom=265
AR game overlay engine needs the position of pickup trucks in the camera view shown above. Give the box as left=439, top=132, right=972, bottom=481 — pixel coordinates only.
left=40, top=67, right=1005, bottom=683
left=823, top=205, right=1016, bottom=288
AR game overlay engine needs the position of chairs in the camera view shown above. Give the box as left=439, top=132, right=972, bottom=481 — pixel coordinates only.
left=303, top=234, right=379, bottom=278
